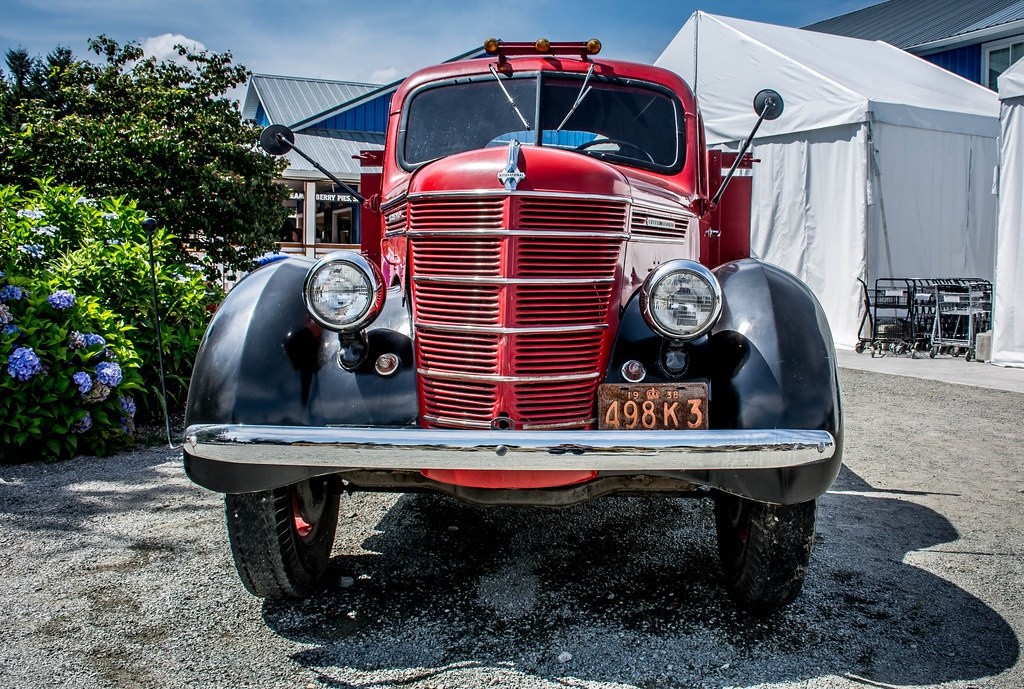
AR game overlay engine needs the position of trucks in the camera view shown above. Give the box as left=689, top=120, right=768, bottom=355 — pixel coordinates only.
left=182, top=39, right=841, bottom=611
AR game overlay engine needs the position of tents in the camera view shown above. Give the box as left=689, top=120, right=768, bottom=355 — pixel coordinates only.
left=988, top=54, right=1024, bottom=368
left=651, top=10, right=1002, bottom=353
